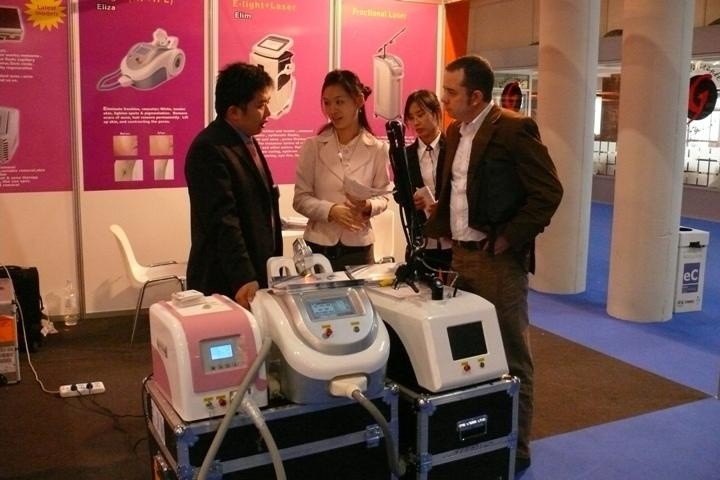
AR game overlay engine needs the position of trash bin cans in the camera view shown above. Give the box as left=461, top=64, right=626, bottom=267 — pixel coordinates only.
left=674, top=226, right=709, bottom=313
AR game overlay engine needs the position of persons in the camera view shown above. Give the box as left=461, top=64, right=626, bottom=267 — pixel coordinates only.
left=183, top=61, right=284, bottom=310
left=291, top=69, right=392, bottom=273
left=419, top=53, right=564, bottom=478
left=393, top=88, right=454, bottom=285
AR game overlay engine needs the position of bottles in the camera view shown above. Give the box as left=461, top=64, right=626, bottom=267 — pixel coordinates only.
left=64, top=280, right=78, bottom=326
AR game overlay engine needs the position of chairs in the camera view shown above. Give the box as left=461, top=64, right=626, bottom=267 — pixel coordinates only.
left=109, top=222, right=190, bottom=350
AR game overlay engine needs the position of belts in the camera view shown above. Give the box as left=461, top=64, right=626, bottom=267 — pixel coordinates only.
left=452, top=239, right=487, bottom=249
